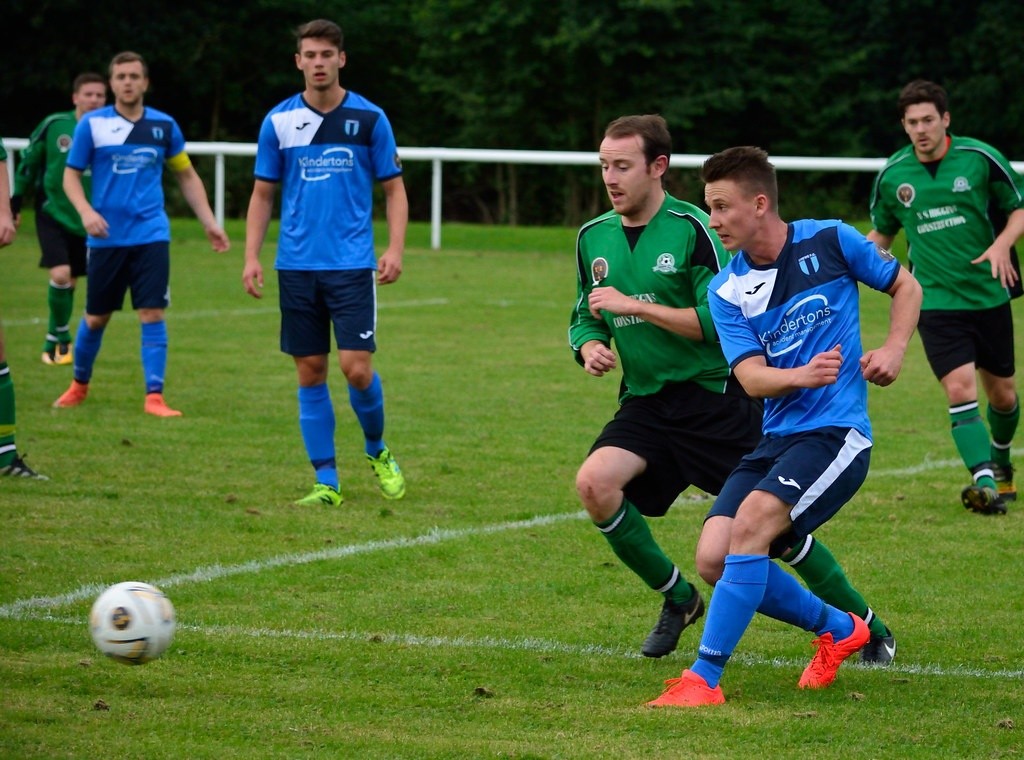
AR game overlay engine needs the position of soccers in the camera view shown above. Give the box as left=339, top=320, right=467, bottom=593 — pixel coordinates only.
left=89, top=581, right=177, bottom=665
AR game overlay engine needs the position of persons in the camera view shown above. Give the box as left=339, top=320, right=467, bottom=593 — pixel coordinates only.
left=0, top=18, right=1024, bottom=709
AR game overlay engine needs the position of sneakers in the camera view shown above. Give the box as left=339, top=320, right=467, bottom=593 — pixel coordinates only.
left=796, top=612, right=871, bottom=690
left=645, top=668, right=726, bottom=707
left=858, top=625, right=897, bottom=667
left=992, top=460, right=1016, bottom=500
left=41, top=352, right=55, bottom=365
left=52, top=379, right=88, bottom=408
left=144, top=393, right=182, bottom=416
left=365, top=447, right=405, bottom=499
left=294, top=482, right=344, bottom=508
left=0, top=455, right=50, bottom=480
left=641, top=582, right=705, bottom=658
left=54, top=342, right=73, bottom=364
left=962, top=485, right=1008, bottom=515
